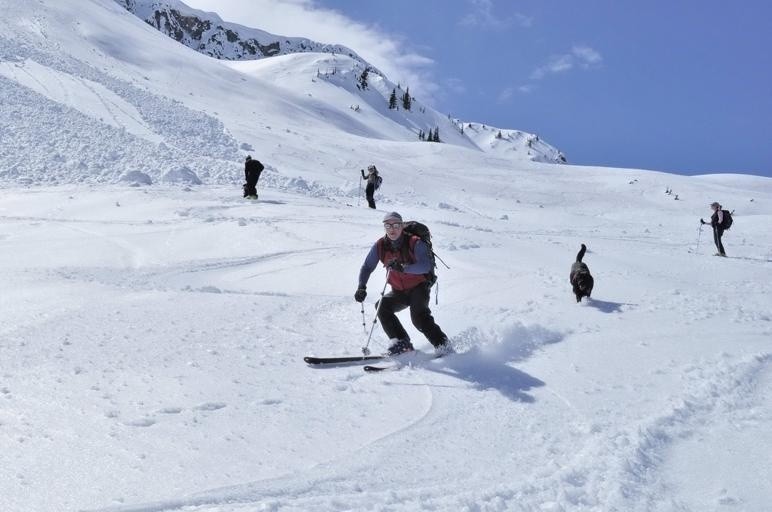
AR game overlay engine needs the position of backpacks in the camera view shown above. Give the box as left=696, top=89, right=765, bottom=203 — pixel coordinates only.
left=403, top=221, right=437, bottom=288
left=722, top=210, right=732, bottom=229
left=374, top=176, right=382, bottom=189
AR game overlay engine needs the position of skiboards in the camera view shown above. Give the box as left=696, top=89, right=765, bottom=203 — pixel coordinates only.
left=688, top=252, right=751, bottom=259
left=304, top=343, right=459, bottom=371
left=346, top=204, right=392, bottom=213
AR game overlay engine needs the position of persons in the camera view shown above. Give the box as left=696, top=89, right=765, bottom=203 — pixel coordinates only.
left=701, top=201, right=726, bottom=256
left=354, top=211, right=451, bottom=358
left=361, top=165, right=378, bottom=209
left=244, top=156, right=264, bottom=199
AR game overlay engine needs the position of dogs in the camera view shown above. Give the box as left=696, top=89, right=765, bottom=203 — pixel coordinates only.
left=570, top=243, right=594, bottom=303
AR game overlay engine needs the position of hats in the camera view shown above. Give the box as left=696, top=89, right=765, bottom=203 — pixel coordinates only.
left=711, top=202, right=719, bottom=206
left=383, top=212, right=402, bottom=222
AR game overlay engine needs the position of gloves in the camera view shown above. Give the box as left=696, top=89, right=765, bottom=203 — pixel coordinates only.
left=387, top=259, right=403, bottom=272
left=355, top=288, right=366, bottom=302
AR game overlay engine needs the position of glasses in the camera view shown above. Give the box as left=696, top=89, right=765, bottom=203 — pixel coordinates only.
left=384, top=223, right=400, bottom=229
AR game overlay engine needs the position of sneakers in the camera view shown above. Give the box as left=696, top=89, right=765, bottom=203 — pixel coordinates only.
left=434, top=345, right=453, bottom=358
left=387, top=339, right=413, bottom=353
